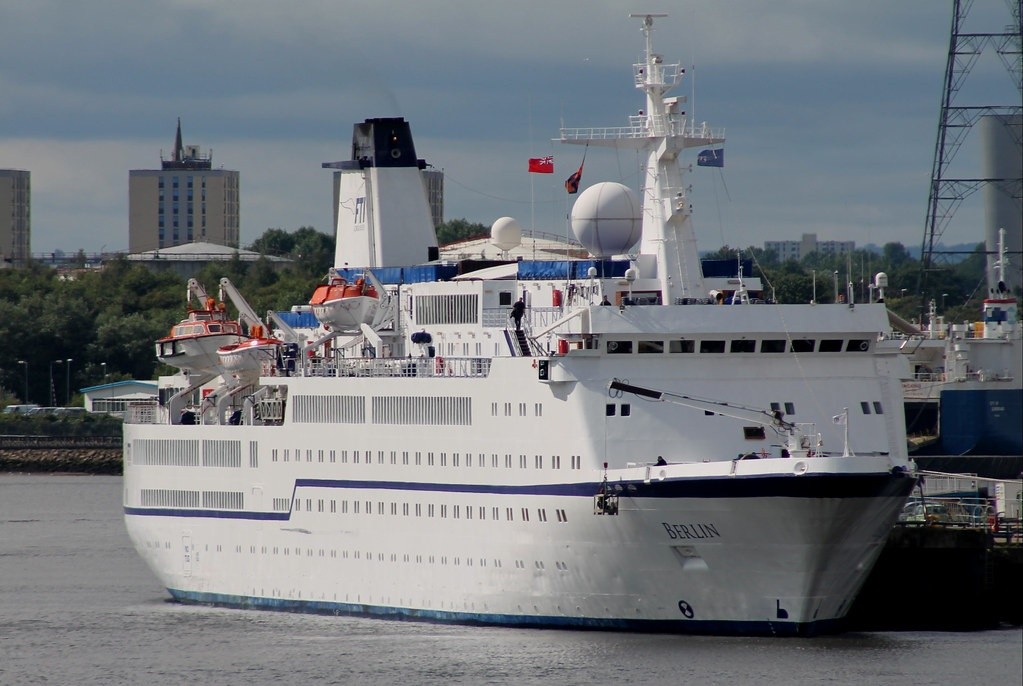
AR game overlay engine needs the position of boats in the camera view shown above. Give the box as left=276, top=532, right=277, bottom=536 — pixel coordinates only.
left=153, top=297, right=251, bottom=370
left=107, top=7, right=927, bottom=635
left=214, top=323, right=285, bottom=372
left=308, top=280, right=382, bottom=331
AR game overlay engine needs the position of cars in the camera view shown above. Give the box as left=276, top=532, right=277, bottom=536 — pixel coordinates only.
left=906, top=503, right=953, bottom=527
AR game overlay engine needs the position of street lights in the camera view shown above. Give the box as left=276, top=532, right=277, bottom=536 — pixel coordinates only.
left=66, top=358, right=74, bottom=406
left=17, top=360, right=29, bottom=405
left=100, top=363, right=106, bottom=384
left=49, top=360, right=62, bottom=407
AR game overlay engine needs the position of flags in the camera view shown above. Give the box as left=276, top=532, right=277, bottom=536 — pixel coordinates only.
left=834, top=412, right=848, bottom=424
left=565, top=163, right=583, bottom=193
left=698, top=149, right=724, bottom=168
left=528, top=156, right=553, bottom=173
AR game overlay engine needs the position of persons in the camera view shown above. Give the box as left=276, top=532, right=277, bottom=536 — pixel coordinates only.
left=969, top=321, right=974, bottom=337
left=837, top=295, right=845, bottom=304
left=181, top=412, right=198, bottom=425
left=601, top=295, right=611, bottom=306
left=230, top=410, right=241, bottom=424
left=510, top=297, right=525, bottom=330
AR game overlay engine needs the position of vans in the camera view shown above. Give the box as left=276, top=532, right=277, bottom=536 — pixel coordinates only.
left=898, top=501, right=944, bottom=521
